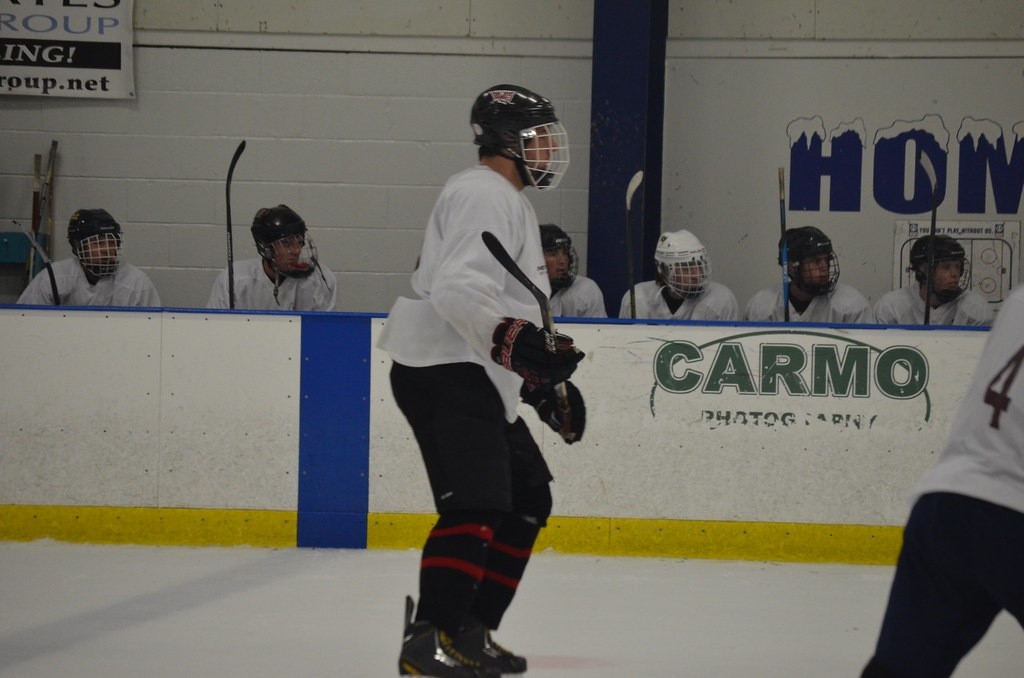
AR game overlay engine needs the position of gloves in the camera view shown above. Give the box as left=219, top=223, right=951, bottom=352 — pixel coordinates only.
left=521, top=375, right=586, bottom=445
left=492, top=315, right=584, bottom=388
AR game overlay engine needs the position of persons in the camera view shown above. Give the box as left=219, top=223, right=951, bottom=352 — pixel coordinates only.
left=746, top=225, right=875, bottom=328
left=620, top=230, right=739, bottom=322
left=14, top=206, right=163, bottom=308
left=863, top=286, right=1024, bottom=678
left=206, top=203, right=342, bottom=314
left=376, top=84, right=586, bottom=677
left=540, top=224, right=609, bottom=319
left=876, top=235, right=994, bottom=328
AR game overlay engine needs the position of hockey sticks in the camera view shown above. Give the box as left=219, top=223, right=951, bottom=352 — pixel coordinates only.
left=224, top=139, right=247, bottom=312
left=626, top=172, right=645, bottom=319
left=779, top=167, right=788, bottom=322
left=42, top=140, right=59, bottom=267
left=482, top=229, right=581, bottom=443
left=21, top=154, right=44, bottom=291
left=923, top=190, right=938, bottom=325
left=13, top=220, right=61, bottom=306
left=28, top=153, right=54, bottom=286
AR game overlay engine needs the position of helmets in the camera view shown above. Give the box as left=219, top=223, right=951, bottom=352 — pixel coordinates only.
left=909, top=233, right=966, bottom=286
left=539, top=223, right=572, bottom=246
left=251, top=205, right=307, bottom=259
left=472, top=83, right=558, bottom=146
left=68, top=207, right=122, bottom=247
left=778, top=226, right=834, bottom=280
left=654, top=228, right=707, bottom=281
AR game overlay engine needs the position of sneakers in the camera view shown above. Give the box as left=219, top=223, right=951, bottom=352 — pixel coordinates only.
left=454, top=623, right=528, bottom=673
left=399, top=596, right=501, bottom=678
